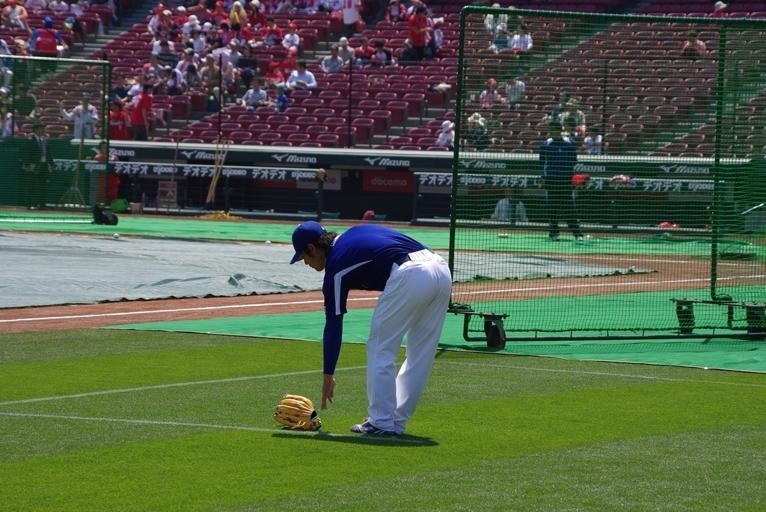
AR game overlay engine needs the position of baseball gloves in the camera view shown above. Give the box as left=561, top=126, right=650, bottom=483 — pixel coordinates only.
left=273, top=393, right=322, bottom=431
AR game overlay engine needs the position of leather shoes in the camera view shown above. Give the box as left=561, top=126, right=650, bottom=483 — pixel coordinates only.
left=29, top=206, right=54, bottom=210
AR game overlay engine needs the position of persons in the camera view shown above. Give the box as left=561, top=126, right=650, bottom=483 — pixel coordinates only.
left=20, top=122, right=57, bottom=210
left=0, top=0, right=529, bottom=152
left=91, top=141, right=121, bottom=208
left=681, top=31, right=706, bottom=56
left=491, top=183, right=528, bottom=222
left=709, top=1, right=728, bottom=17
left=290, top=221, right=452, bottom=438
left=539, top=90, right=603, bottom=241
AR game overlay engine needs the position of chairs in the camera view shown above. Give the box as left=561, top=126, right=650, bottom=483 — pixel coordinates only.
left=0, top=1, right=766, bottom=168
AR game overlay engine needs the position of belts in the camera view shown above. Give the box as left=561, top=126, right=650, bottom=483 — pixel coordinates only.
left=395, top=249, right=436, bottom=266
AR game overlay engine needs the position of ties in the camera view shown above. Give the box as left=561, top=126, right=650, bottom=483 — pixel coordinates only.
left=39, top=137, right=44, bottom=156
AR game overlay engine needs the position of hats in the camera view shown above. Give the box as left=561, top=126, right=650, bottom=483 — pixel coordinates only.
left=80, top=92, right=91, bottom=98
left=290, top=220, right=327, bottom=264
left=497, top=23, right=508, bottom=33
left=565, top=97, right=579, bottom=107
left=42, top=17, right=54, bottom=25
left=149, top=0, right=427, bottom=72
left=442, top=111, right=485, bottom=131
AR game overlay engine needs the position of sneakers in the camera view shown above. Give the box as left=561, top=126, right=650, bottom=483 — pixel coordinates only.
left=576, top=236, right=583, bottom=241
left=350, top=417, right=402, bottom=436
left=552, top=236, right=560, bottom=240
